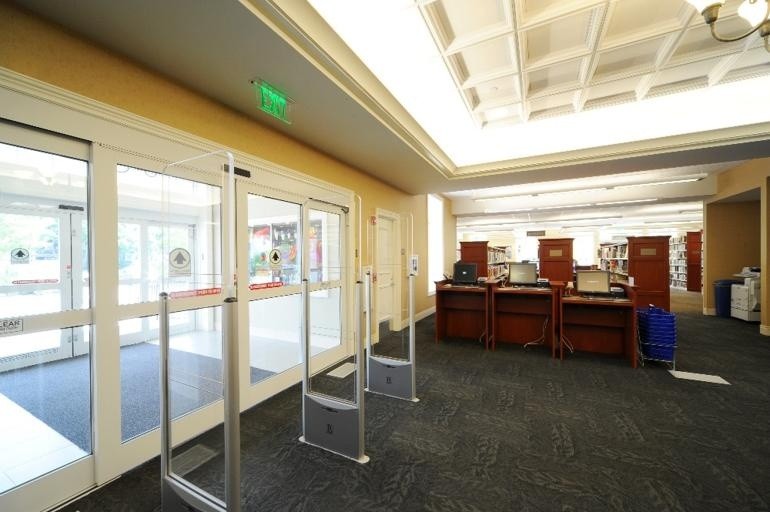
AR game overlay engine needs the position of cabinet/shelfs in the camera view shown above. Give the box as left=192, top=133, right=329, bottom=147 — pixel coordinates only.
left=539, top=239, right=573, bottom=284
left=601, top=231, right=702, bottom=311
left=460, top=241, right=507, bottom=280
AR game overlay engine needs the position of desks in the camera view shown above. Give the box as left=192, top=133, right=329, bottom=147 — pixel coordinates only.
left=434, top=277, right=638, bottom=369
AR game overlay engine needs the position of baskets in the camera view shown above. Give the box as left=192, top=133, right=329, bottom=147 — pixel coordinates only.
left=637, top=308, right=677, bottom=361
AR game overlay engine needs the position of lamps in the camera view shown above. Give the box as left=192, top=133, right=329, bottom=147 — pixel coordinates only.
left=682, top=0, right=770, bottom=50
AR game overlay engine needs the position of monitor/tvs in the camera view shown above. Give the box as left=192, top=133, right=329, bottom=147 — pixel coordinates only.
left=452, top=263, right=477, bottom=285
left=575, top=270, right=611, bottom=298
left=508, top=262, right=537, bottom=287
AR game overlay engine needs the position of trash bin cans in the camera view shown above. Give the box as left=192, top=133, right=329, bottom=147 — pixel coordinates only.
left=715, top=280, right=744, bottom=316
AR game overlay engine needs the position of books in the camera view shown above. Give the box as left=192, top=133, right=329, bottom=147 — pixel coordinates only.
left=487, top=248, right=510, bottom=276
left=669, top=237, right=690, bottom=290
left=596, top=244, right=628, bottom=280
